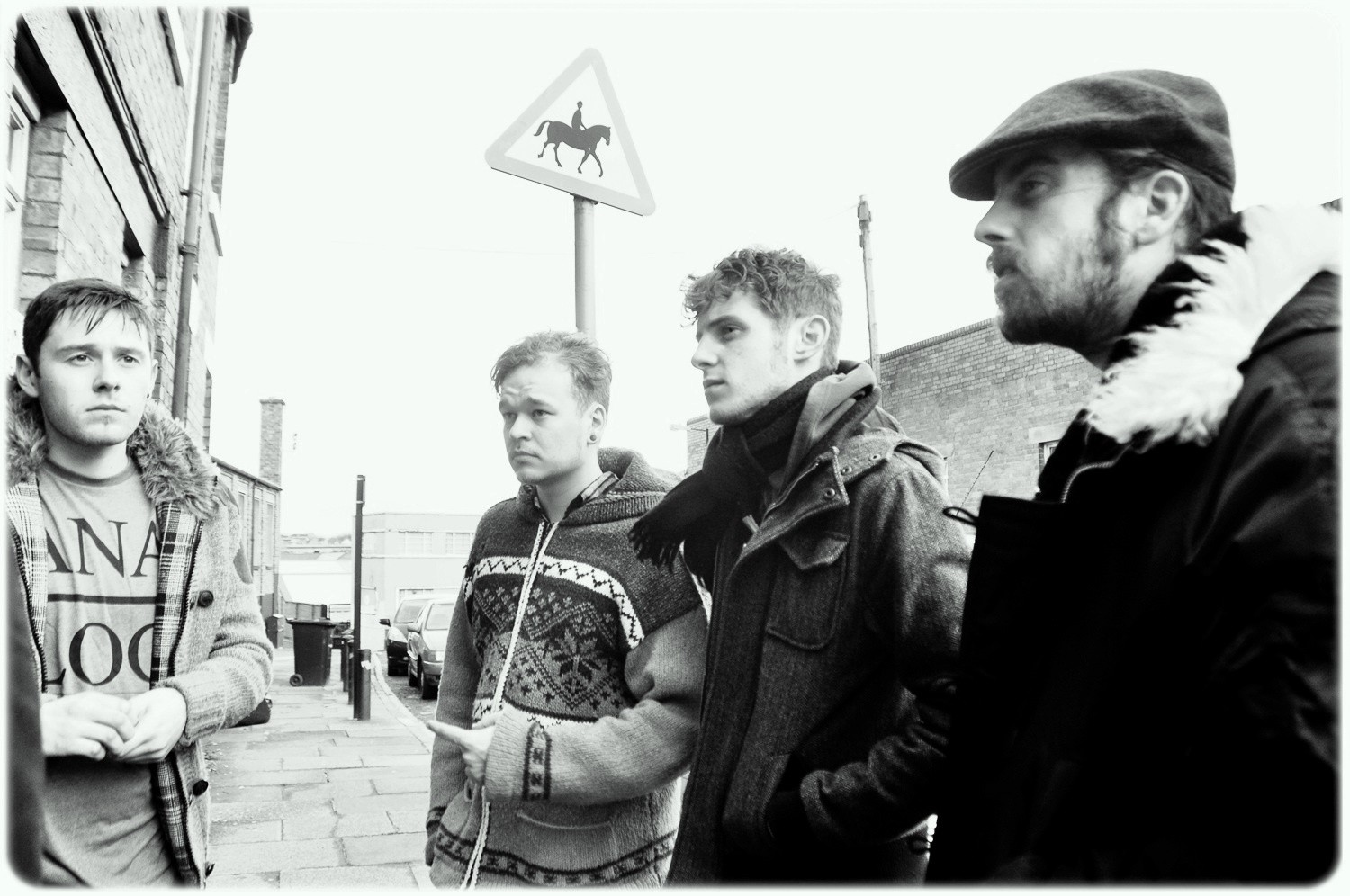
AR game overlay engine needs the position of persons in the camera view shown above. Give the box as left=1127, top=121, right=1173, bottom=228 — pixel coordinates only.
left=925, top=70, right=1341, bottom=886
left=425, top=332, right=708, bottom=887
left=7, top=278, right=273, bottom=888
left=662, top=246, right=965, bottom=887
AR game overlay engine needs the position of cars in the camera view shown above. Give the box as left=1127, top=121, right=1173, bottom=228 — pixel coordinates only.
left=406, top=598, right=456, bottom=700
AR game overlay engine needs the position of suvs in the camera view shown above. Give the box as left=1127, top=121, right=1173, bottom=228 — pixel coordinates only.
left=378, top=595, right=434, bottom=677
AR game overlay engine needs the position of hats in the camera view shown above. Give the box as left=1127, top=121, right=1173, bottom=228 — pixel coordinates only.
left=949, top=69, right=1235, bottom=201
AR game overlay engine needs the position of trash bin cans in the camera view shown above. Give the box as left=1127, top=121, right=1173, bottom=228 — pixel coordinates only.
left=287, top=618, right=337, bottom=686
